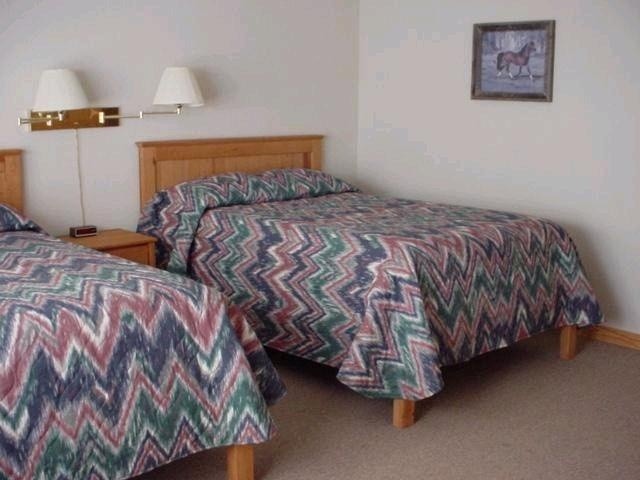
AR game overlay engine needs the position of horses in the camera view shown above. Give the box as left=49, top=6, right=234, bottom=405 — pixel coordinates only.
left=496, top=41, right=537, bottom=80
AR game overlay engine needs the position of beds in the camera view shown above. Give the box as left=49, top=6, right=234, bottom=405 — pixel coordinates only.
left=131, top=129, right=605, bottom=429
left=0, top=147, right=291, bottom=479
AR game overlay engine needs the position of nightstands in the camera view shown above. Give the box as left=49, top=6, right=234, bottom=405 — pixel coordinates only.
left=50, top=222, right=161, bottom=272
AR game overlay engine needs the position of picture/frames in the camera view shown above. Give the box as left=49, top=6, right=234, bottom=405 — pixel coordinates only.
left=465, top=14, right=556, bottom=106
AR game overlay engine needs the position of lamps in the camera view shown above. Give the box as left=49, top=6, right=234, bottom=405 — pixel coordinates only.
left=12, top=59, right=208, bottom=133
